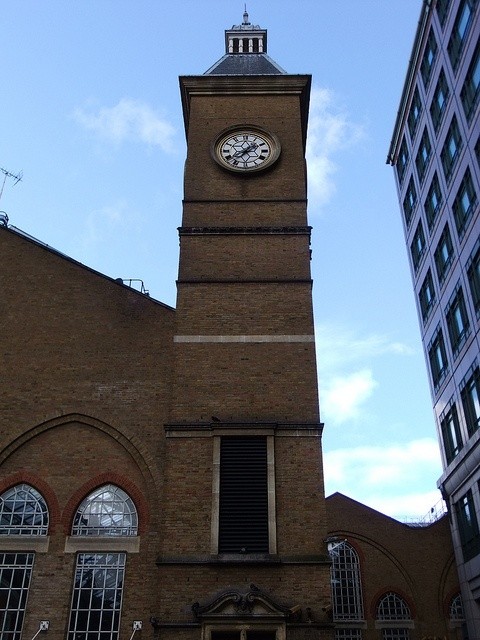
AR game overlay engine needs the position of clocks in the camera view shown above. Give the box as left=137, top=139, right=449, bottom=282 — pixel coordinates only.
left=210, top=122, right=281, bottom=174
